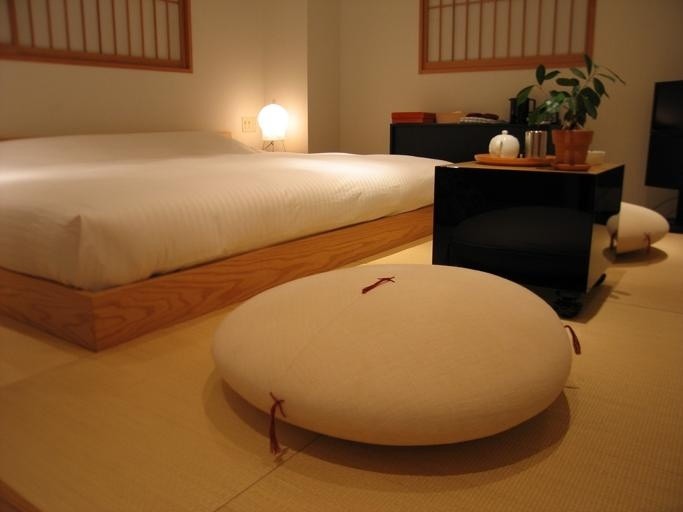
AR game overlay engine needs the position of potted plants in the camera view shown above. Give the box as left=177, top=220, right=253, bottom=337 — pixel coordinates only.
left=514, top=50, right=627, bottom=164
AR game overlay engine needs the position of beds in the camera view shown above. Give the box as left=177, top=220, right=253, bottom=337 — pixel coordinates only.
left=0, top=130, right=456, bottom=353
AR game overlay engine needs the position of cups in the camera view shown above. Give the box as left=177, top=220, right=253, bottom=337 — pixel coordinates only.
left=524, top=131, right=547, bottom=158
left=585, top=150, right=605, bottom=165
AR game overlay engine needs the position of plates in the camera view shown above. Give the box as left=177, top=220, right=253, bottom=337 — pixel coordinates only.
left=474, top=154, right=557, bottom=167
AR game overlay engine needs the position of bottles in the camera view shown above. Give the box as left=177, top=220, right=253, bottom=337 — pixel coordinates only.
left=489, top=130, right=520, bottom=158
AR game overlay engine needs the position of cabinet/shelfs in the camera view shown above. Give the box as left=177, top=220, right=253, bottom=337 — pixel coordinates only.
left=432, top=155, right=626, bottom=321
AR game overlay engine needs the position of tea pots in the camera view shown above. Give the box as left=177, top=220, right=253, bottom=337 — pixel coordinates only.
left=509, top=98, right=536, bottom=126
